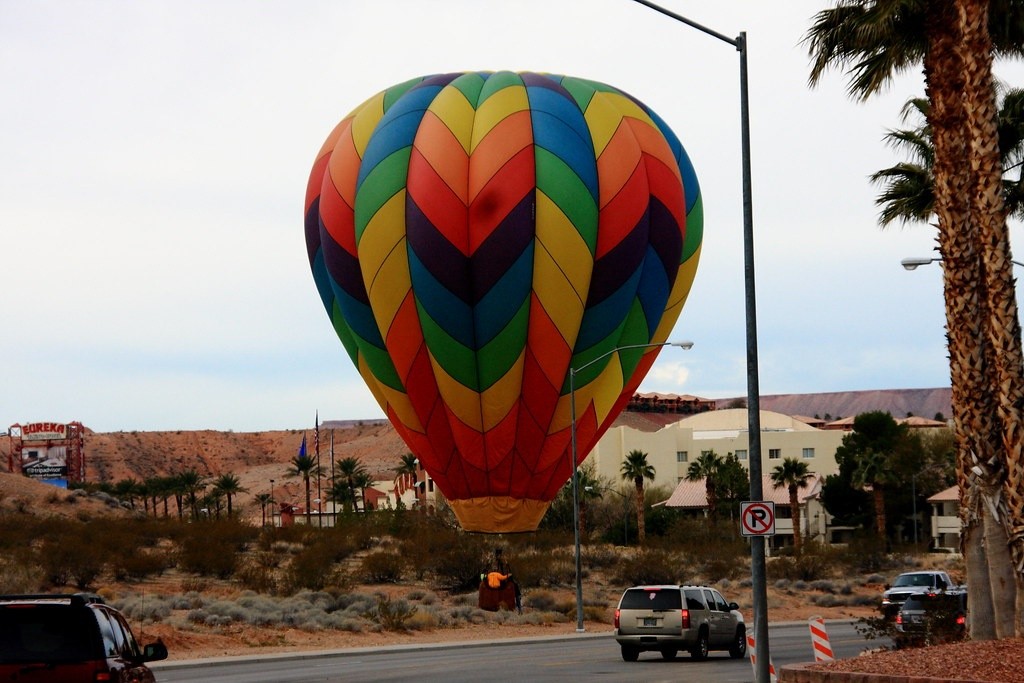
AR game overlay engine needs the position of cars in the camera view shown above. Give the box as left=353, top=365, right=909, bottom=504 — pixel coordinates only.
left=895, top=591, right=968, bottom=648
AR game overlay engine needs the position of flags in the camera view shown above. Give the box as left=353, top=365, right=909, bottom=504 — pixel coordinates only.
left=329, top=436, right=332, bottom=464
left=314, top=430, right=319, bottom=459
left=298, top=439, right=306, bottom=457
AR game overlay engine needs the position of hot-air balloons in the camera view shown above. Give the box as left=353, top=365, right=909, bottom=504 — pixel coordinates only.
left=304, top=70, right=703, bottom=612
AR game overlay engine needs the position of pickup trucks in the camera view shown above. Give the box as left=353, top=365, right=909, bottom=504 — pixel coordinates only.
left=882, top=571, right=968, bottom=618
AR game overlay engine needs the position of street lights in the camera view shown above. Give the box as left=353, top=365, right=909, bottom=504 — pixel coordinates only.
left=570, top=341, right=694, bottom=629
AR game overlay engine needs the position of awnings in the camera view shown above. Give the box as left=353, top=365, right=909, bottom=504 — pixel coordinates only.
left=415, top=481, right=425, bottom=487
left=414, top=459, right=420, bottom=463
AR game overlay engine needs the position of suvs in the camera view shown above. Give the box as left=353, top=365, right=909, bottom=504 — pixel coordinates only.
left=0, top=592, right=168, bottom=683
left=613, top=584, right=747, bottom=661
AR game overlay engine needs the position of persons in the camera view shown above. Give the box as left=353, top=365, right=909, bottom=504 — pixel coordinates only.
left=487, top=566, right=512, bottom=589
